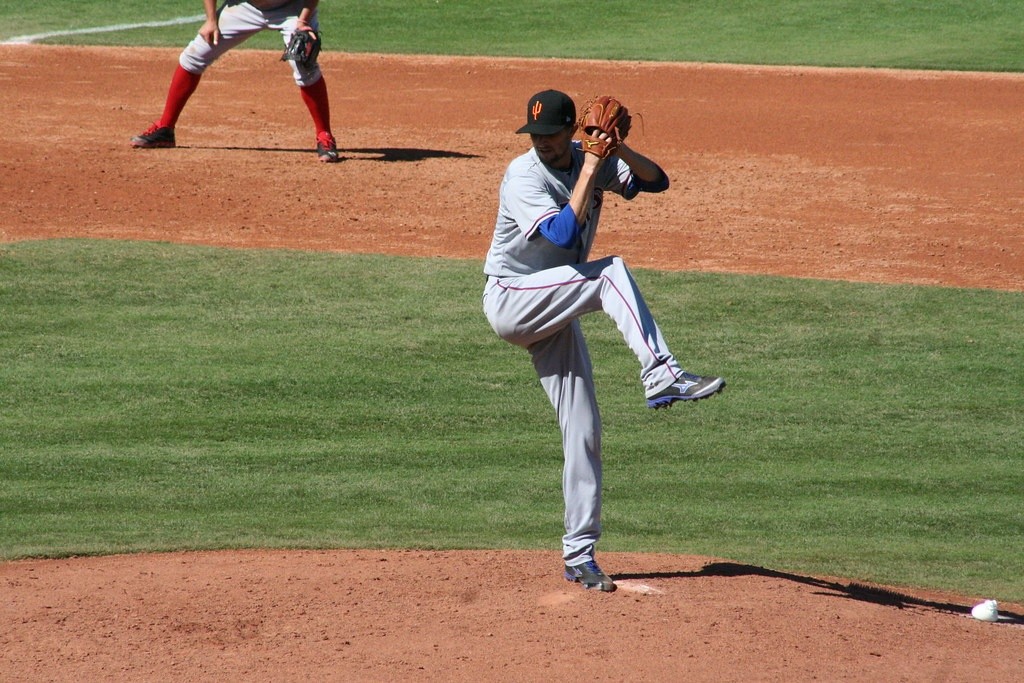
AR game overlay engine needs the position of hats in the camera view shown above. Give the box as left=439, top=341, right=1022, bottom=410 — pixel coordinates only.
left=516, top=89, right=577, bottom=135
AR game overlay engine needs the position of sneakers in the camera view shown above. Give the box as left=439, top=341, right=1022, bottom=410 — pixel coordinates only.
left=646, top=371, right=727, bottom=411
left=565, top=559, right=618, bottom=592
left=129, top=121, right=178, bottom=147
left=315, top=132, right=339, bottom=163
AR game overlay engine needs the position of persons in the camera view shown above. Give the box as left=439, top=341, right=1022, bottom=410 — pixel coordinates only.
left=482, top=89, right=726, bottom=591
left=130, top=0, right=339, bottom=161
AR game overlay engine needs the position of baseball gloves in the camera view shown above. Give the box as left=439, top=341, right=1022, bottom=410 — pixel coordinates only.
left=279, top=25, right=324, bottom=71
left=572, top=89, right=646, bottom=163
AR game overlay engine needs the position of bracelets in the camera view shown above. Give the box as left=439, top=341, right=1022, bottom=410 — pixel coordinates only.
left=297, top=20, right=308, bottom=24
